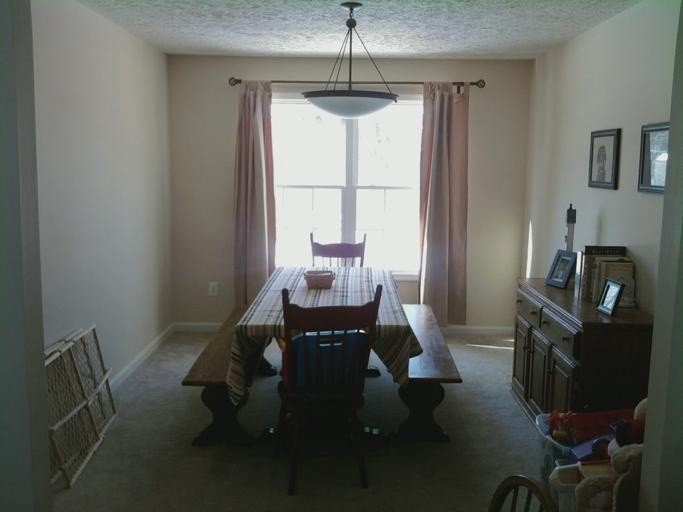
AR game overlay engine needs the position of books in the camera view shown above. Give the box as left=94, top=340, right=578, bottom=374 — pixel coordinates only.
left=574, top=244, right=639, bottom=309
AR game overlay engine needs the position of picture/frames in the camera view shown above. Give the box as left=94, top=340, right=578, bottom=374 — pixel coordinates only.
left=545, top=249, right=576, bottom=289
left=595, top=279, right=624, bottom=316
left=638, top=123, right=671, bottom=193
left=587, top=127, right=620, bottom=194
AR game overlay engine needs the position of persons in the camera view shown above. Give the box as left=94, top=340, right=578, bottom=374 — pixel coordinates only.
left=596, top=145, right=607, bottom=181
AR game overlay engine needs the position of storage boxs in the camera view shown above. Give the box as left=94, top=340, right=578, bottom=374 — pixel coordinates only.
left=536, top=410, right=646, bottom=488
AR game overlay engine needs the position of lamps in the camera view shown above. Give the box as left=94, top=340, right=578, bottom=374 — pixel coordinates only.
left=303, top=3, right=400, bottom=119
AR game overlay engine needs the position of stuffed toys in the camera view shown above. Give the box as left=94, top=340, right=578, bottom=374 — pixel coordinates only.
left=568, top=439, right=643, bottom=510
left=543, top=397, right=648, bottom=461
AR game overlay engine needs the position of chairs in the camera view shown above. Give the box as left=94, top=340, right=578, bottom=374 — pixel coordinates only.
left=271, top=285, right=382, bottom=495
left=311, top=232, right=366, bottom=267
left=486, top=475, right=559, bottom=511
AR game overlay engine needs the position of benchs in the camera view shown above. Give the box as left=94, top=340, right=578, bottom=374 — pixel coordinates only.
left=182, top=304, right=277, bottom=447
left=386, top=299, right=463, bottom=442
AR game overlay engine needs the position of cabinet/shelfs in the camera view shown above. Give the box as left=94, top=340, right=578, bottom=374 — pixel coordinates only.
left=510, top=278, right=654, bottom=431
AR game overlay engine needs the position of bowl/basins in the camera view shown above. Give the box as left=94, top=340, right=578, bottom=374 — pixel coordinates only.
left=303, top=269, right=335, bottom=290
